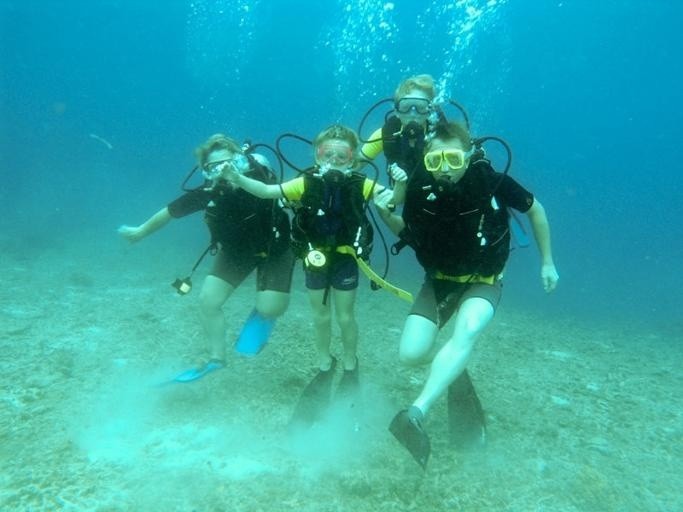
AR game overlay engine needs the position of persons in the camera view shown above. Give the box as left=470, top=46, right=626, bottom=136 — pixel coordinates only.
left=213, top=124, right=407, bottom=426
left=372, top=122, right=562, bottom=470
left=114, top=130, right=296, bottom=383
left=350, top=71, right=454, bottom=241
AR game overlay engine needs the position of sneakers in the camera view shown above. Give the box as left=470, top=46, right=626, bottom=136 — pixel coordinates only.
left=318, top=356, right=336, bottom=374
left=208, top=358, right=225, bottom=368
left=342, top=356, right=358, bottom=377
left=408, top=406, right=423, bottom=422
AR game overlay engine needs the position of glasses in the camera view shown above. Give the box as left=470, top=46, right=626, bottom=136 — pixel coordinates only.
left=318, top=145, right=351, bottom=164
left=395, top=99, right=430, bottom=115
left=204, top=160, right=234, bottom=173
left=424, top=146, right=476, bottom=172
